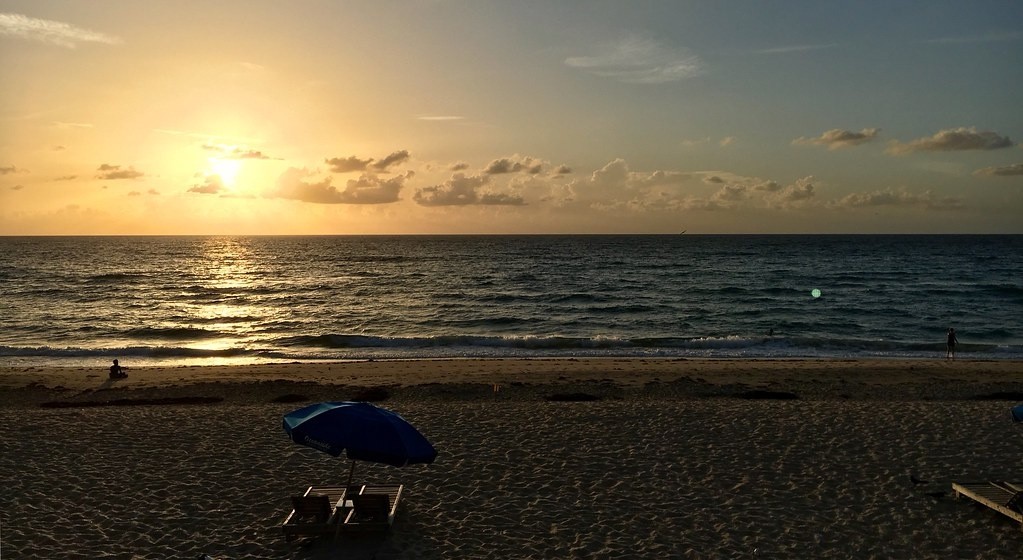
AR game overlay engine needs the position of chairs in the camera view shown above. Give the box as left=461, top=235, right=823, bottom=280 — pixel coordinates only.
left=952, top=480, right=1023, bottom=533
left=282, top=485, right=347, bottom=542
left=344, top=484, right=404, bottom=540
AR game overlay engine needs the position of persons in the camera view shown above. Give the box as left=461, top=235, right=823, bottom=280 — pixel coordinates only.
left=946, top=328, right=958, bottom=357
left=108, top=359, right=128, bottom=379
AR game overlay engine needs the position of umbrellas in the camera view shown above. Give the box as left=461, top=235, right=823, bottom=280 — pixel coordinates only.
left=281, top=401, right=438, bottom=542
